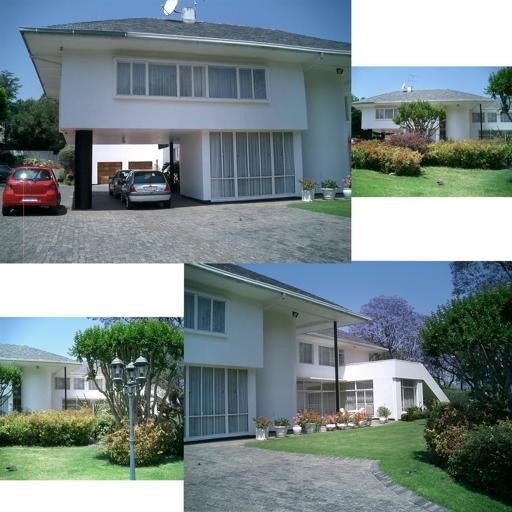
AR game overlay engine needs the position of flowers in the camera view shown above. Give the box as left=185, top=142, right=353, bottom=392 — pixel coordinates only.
left=250, top=404, right=392, bottom=440
left=342, top=174, right=352, bottom=188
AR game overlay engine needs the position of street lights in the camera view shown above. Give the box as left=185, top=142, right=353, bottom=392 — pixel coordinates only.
left=109, top=346, right=150, bottom=481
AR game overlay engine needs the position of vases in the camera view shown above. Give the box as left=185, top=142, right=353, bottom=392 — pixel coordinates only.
left=342, top=187, right=352, bottom=199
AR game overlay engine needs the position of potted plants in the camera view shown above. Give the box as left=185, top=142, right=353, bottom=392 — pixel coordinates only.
left=297, top=175, right=318, bottom=202
left=319, top=178, right=341, bottom=200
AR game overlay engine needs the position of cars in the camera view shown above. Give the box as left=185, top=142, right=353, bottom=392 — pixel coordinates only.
left=105, top=162, right=180, bottom=208
left=1, top=162, right=64, bottom=214
